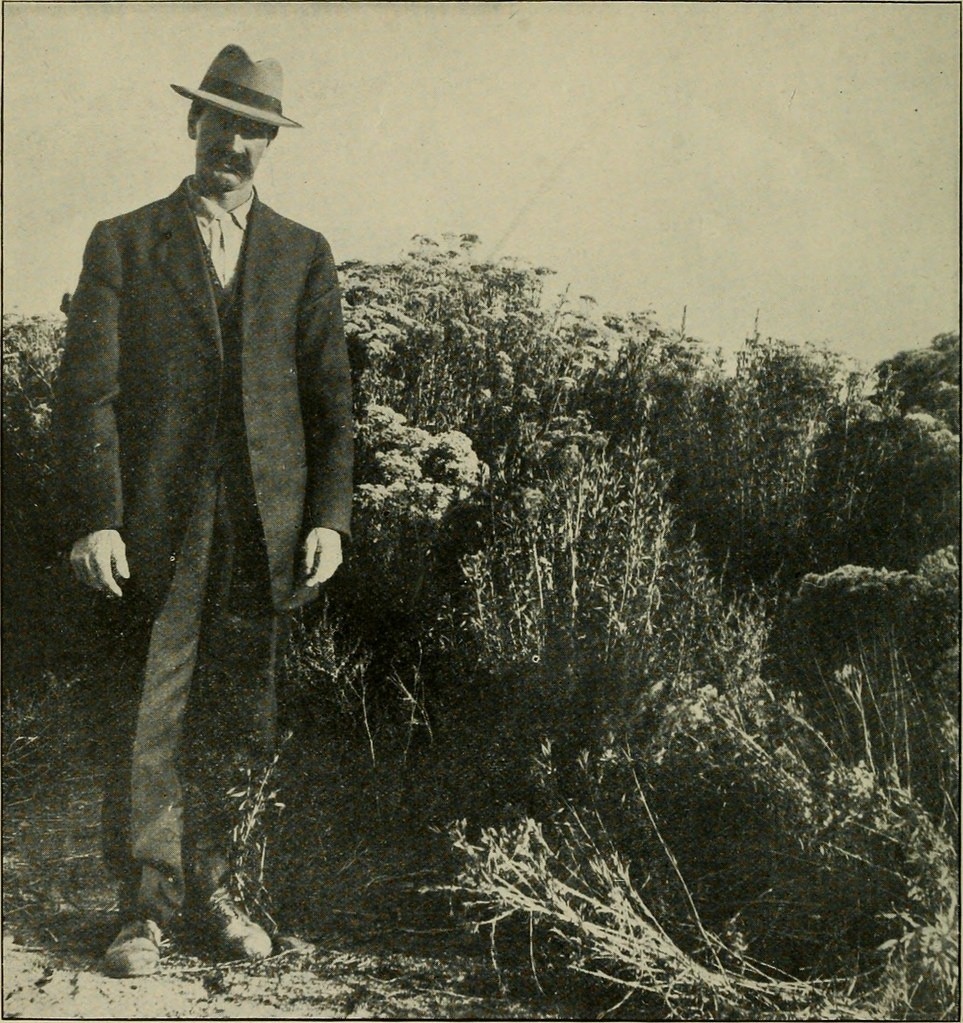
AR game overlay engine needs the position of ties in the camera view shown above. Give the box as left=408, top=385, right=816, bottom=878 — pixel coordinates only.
left=209, top=212, right=239, bottom=286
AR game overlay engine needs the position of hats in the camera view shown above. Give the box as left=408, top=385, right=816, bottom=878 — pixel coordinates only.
left=169, top=43, right=302, bottom=129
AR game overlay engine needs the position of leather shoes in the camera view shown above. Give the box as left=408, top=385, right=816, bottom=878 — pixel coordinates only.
left=104, top=919, right=163, bottom=978
left=180, top=886, right=272, bottom=960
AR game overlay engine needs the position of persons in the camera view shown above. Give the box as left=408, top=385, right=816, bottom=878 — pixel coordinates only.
left=51, top=46, right=356, bottom=979
